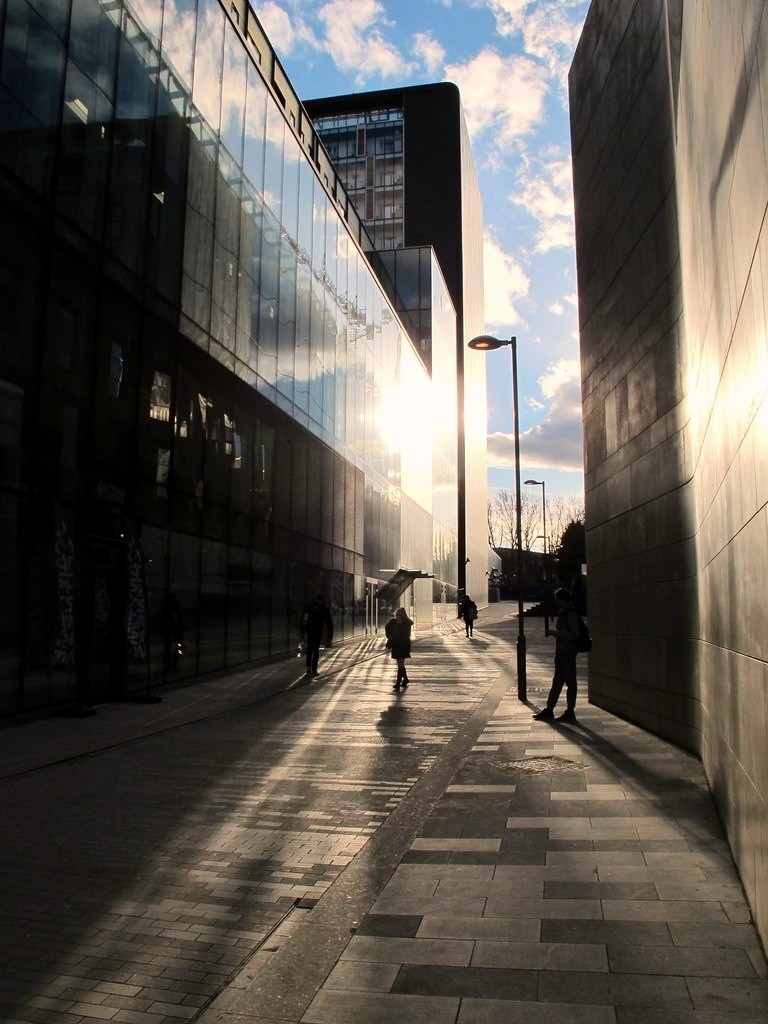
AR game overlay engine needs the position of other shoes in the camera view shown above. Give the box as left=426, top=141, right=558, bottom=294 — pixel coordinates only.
left=555, top=711, right=576, bottom=721
left=532, top=708, right=554, bottom=719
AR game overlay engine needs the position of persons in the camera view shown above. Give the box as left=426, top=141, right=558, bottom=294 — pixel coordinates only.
left=532, top=587, right=582, bottom=722
left=300, top=593, right=334, bottom=676
left=385, top=607, right=414, bottom=688
left=459, top=594, right=477, bottom=637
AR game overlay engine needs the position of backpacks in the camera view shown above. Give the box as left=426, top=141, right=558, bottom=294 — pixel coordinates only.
left=566, top=609, right=592, bottom=653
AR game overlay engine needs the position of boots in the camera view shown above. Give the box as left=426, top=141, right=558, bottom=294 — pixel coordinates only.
left=393, top=667, right=404, bottom=688
left=402, top=667, right=409, bottom=686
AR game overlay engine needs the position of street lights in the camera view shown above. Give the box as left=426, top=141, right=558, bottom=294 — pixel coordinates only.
left=469, top=336, right=526, bottom=703
left=523, top=478, right=548, bottom=601
